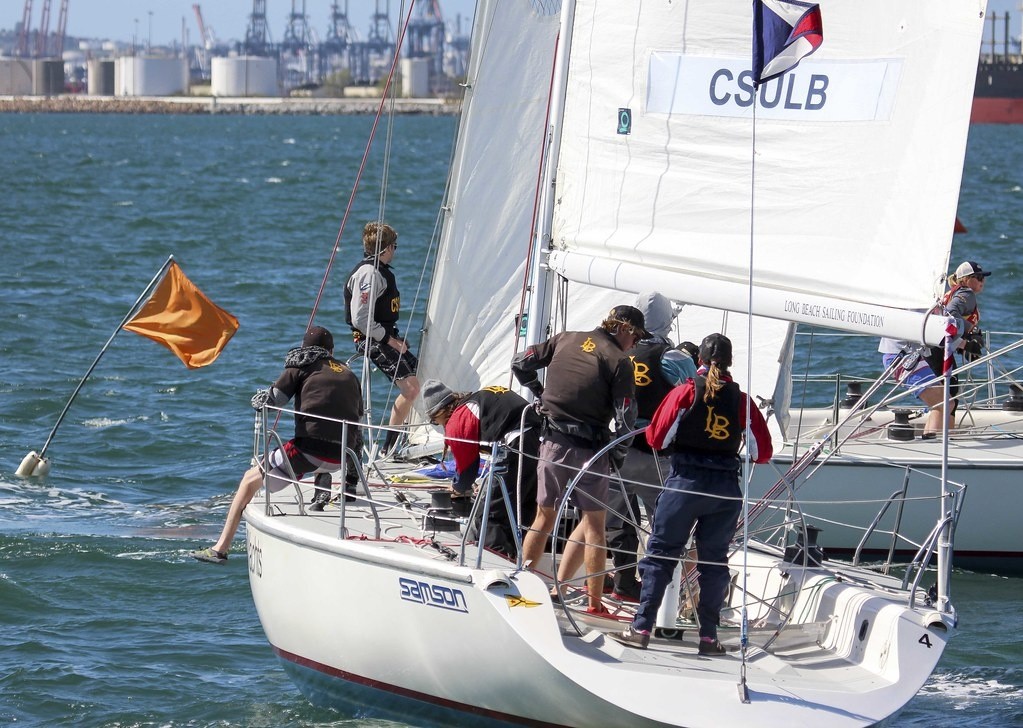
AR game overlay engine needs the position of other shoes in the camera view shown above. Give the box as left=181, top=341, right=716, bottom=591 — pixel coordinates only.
left=588, top=603, right=617, bottom=619
left=378, top=447, right=402, bottom=462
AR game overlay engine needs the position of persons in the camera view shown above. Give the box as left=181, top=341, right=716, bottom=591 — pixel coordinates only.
left=925, top=262, right=993, bottom=432
left=877, top=337, right=980, bottom=440
left=603, top=293, right=702, bottom=605
left=188, top=327, right=365, bottom=564
left=343, top=220, right=419, bottom=458
left=510, top=305, right=656, bottom=624
left=419, top=378, right=541, bottom=559
left=606, top=331, right=773, bottom=655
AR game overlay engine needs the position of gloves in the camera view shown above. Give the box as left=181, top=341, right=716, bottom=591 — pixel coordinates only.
left=345, top=483, right=357, bottom=502
left=959, top=348, right=979, bottom=362
left=965, top=341, right=981, bottom=354
left=609, top=450, right=627, bottom=473
left=533, top=396, right=543, bottom=415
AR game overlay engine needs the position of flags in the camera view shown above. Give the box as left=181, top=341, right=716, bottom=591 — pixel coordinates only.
left=121, top=259, right=240, bottom=370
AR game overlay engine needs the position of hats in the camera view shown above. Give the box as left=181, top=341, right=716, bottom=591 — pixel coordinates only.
left=700, top=333, right=732, bottom=363
left=608, top=305, right=654, bottom=339
left=955, top=261, right=991, bottom=279
left=303, top=326, right=334, bottom=350
left=676, top=341, right=700, bottom=363
left=421, top=379, right=457, bottom=417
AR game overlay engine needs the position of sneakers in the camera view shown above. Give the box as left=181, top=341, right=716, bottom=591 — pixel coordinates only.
left=188, top=547, right=227, bottom=565
left=697, top=639, right=726, bottom=655
left=311, top=473, right=332, bottom=507
left=607, top=625, right=649, bottom=648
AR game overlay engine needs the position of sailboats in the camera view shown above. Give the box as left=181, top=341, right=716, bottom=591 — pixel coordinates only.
left=239, top=1, right=992, bottom=728
left=392, top=1, right=1023, bottom=566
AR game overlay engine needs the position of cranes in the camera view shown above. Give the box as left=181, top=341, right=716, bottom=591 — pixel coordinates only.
left=232, top=0, right=447, bottom=95
left=5, top=0, right=68, bottom=60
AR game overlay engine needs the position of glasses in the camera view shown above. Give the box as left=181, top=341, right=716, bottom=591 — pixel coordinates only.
left=974, top=275, right=984, bottom=281
left=629, top=327, right=641, bottom=344
left=429, top=412, right=439, bottom=426
left=390, top=243, right=397, bottom=249
